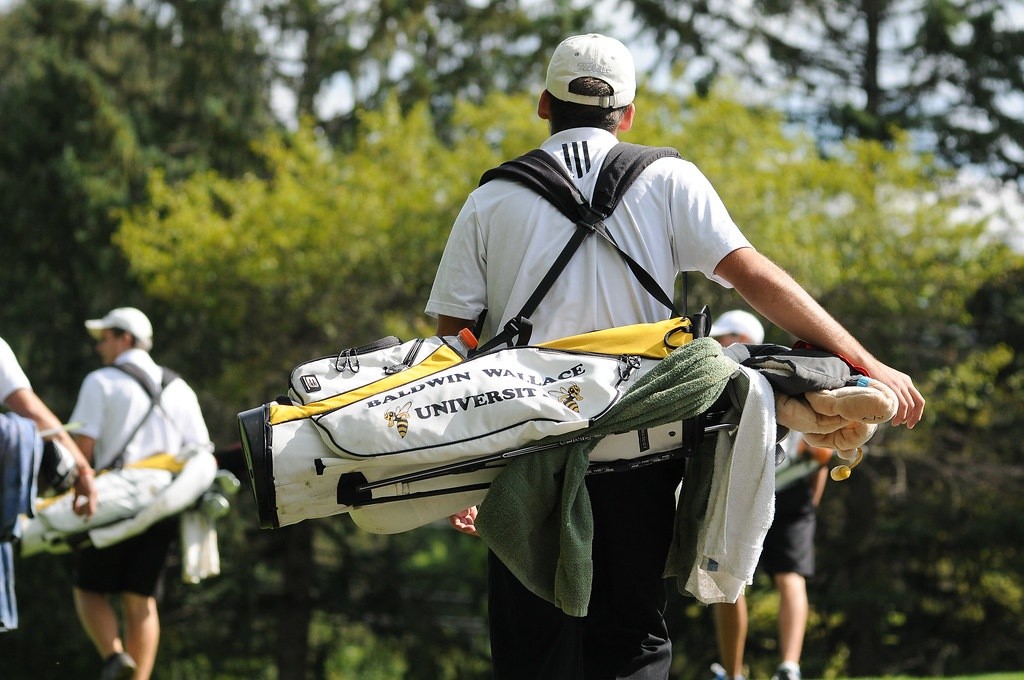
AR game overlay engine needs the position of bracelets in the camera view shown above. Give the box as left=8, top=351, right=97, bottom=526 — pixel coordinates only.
left=79, top=468, right=97, bottom=476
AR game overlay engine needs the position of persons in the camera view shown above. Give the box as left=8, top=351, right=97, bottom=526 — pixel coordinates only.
left=54, top=305, right=215, bottom=680
left=0, top=336, right=98, bottom=523
left=707, top=309, right=828, bottom=680
left=423, top=35, right=924, bottom=680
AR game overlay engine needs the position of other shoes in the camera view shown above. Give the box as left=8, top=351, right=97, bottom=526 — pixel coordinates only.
left=771, top=669, right=800, bottom=680
left=104, top=652, right=137, bottom=680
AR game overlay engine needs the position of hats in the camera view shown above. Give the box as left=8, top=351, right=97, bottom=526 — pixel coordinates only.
left=546, top=33, right=636, bottom=109
left=84, top=307, right=154, bottom=349
left=709, top=310, right=765, bottom=344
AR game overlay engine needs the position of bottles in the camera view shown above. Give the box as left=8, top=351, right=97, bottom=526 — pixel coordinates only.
left=442, top=327, right=478, bottom=361
